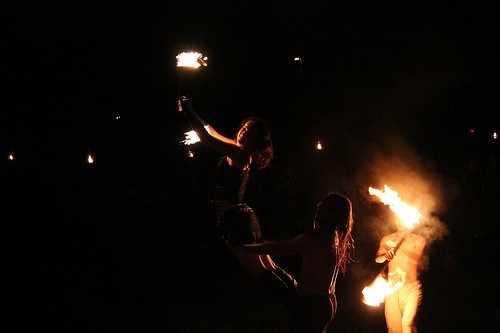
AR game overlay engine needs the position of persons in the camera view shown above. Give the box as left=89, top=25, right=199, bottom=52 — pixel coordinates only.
left=177, top=93, right=301, bottom=312
left=374, top=213, right=427, bottom=333
left=223, top=193, right=353, bottom=333
